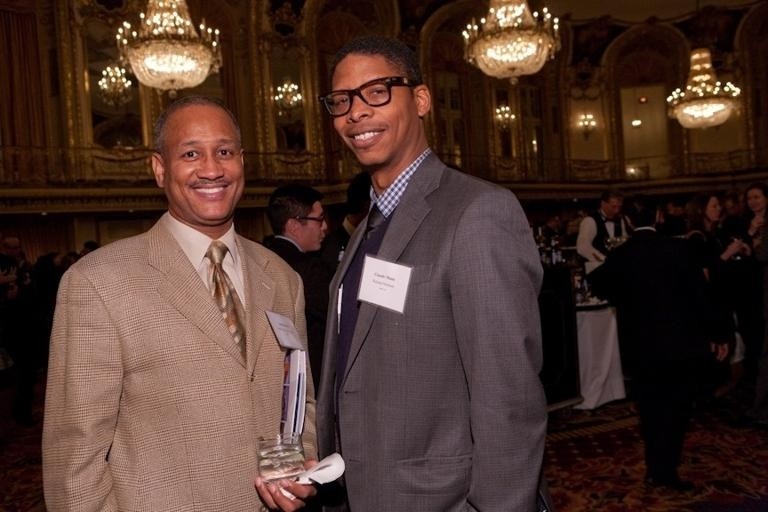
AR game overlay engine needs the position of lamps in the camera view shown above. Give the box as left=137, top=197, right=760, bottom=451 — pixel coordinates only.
left=492, top=103, right=516, bottom=129
left=272, top=79, right=307, bottom=117
left=114, top=1, right=228, bottom=100
left=575, top=112, right=600, bottom=139
left=93, top=64, right=134, bottom=99
left=666, top=44, right=747, bottom=131
left=454, top=1, right=569, bottom=85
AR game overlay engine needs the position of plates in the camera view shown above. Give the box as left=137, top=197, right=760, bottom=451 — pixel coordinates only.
left=576, top=302, right=609, bottom=312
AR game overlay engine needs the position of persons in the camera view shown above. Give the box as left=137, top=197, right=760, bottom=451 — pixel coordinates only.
left=543, top=185, right=766, bottom=484
left=1, top=238, right=99, bottom=424
left=316, top=170, right=373, bottom=308
left=267, top=183, right=329, bottom=287
left=41, top=96, right=316, bottom=510
left=316, top=37, right=546, bottom=511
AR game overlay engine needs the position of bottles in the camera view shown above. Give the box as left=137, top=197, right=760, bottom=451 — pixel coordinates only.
left=576, top=277, right=592, bottom=302
left=535, top=234, right=562, bottom=264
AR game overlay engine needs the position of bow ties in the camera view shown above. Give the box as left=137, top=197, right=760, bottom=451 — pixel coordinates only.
left=604, top=217, right=613, bottom=221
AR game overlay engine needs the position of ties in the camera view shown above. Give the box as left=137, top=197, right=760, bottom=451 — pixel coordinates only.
left=204, top=239, right=246, bottom=366
left=336, top=203, right=385, bottom=454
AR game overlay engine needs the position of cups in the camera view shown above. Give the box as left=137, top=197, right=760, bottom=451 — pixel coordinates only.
left=253, top=432, right=308, bottom=493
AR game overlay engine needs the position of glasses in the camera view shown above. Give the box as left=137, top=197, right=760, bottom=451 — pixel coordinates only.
left=317, top=77, right=415, bottom=117
left=296, top=215, right=327, bottom=227
